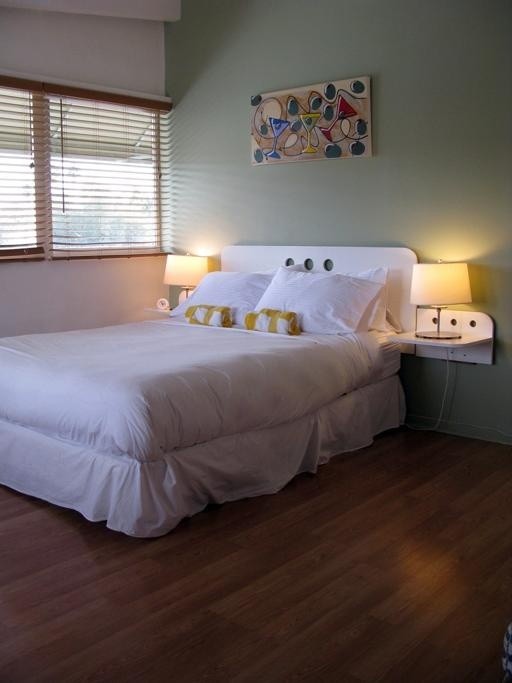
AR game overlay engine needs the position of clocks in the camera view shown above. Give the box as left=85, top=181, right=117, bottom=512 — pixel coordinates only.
left=157, top=299, right=168, bottom=310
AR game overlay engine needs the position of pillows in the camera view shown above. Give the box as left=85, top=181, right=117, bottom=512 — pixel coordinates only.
left=255, top=267, right=383, bottom=330
left=172, top=271, right=273, bottom=325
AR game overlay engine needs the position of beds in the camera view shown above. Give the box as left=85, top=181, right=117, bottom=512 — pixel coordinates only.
left=0, top=244, right=420, bottom=538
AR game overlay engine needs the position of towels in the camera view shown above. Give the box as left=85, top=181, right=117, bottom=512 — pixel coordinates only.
left=185, top=304, right=233, bottom=328
left=245, top=310, right=299, bottom=334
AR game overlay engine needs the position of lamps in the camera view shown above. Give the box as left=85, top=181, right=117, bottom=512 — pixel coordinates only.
left=163, top=253, right=208, bottom=299
left=408, top=258, right=471, bottom=340
